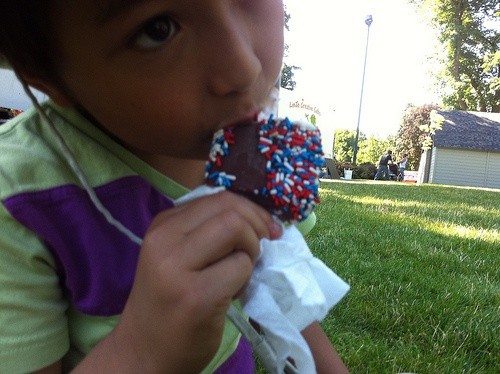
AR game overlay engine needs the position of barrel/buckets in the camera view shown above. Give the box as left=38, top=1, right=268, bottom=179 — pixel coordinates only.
left=344, top=168, right=352, bottom=179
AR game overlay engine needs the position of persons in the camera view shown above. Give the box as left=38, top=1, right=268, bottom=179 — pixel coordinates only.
left=0, top=0, right=350, bottom=374
left=397, top=154, right=408, bottom=181
left=374, top=150, right=394, bottom=180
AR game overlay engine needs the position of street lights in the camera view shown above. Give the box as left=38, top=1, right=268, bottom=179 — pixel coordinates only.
left=351, top=13, right=373, bottom=164
